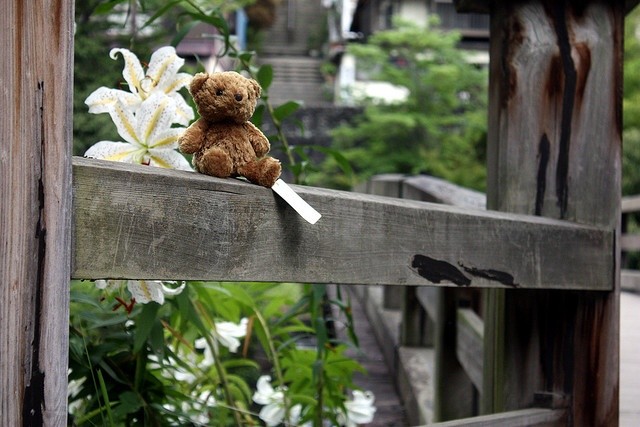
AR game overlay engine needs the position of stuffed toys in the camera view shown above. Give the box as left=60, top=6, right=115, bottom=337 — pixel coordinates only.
left=176, top=69, right=282, bottom=188
